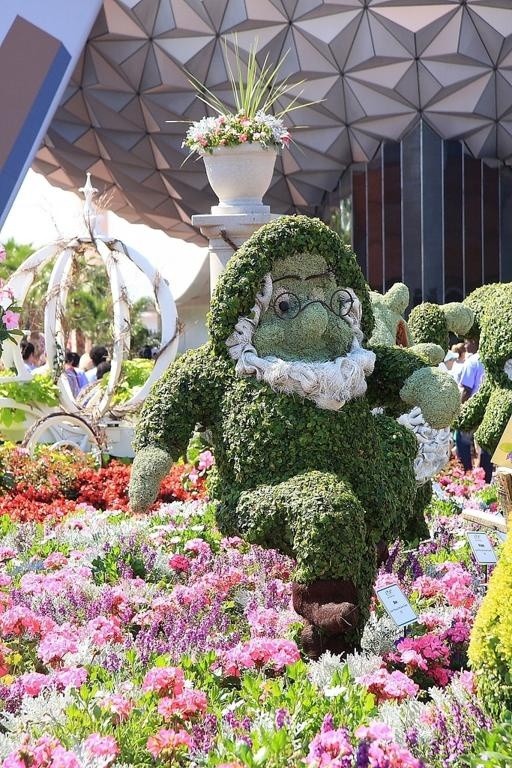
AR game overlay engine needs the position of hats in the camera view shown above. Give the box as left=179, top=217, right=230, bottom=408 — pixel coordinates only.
left=444, top=350, right=460, bottom=362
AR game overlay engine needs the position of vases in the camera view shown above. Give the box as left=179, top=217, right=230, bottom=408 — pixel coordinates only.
left=199, top=142, right=279, bottom=206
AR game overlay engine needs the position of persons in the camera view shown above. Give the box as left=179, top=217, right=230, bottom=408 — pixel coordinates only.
left=15, top=325, right=162, bottom=399
left=436, top=329, right=497, bottom=485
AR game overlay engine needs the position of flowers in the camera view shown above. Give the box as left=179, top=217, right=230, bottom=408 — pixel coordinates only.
left=167, top=31, right=326, bottom=167
